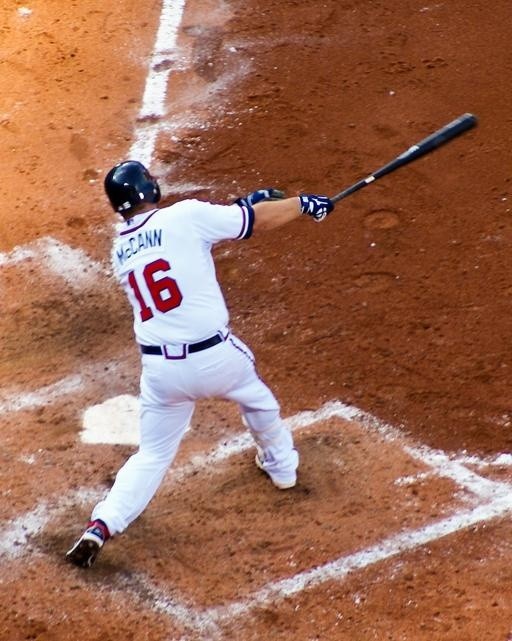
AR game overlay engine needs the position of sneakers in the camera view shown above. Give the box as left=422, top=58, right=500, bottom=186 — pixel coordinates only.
left=64, top=521, right=106, bottom=567
left=254, top=455, right=296, bottom=490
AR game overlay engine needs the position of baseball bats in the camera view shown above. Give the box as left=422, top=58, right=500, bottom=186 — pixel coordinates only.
left=331, top=112, right=477, bottom=206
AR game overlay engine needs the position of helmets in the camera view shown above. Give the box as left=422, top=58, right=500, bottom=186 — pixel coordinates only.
left=103, top=160, right=160, bottom=212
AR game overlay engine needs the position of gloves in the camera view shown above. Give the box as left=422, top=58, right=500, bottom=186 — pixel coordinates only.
left=299, top=194, right=334, bottom=223
left=234, top=189, right=284, bottom=208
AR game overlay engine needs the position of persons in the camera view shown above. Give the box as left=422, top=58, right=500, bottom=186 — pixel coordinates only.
left=62, top=160, right=336, bottom=568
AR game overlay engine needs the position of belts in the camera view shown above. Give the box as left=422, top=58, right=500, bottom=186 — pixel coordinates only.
left=140, top=333, right=222, bottom=355
